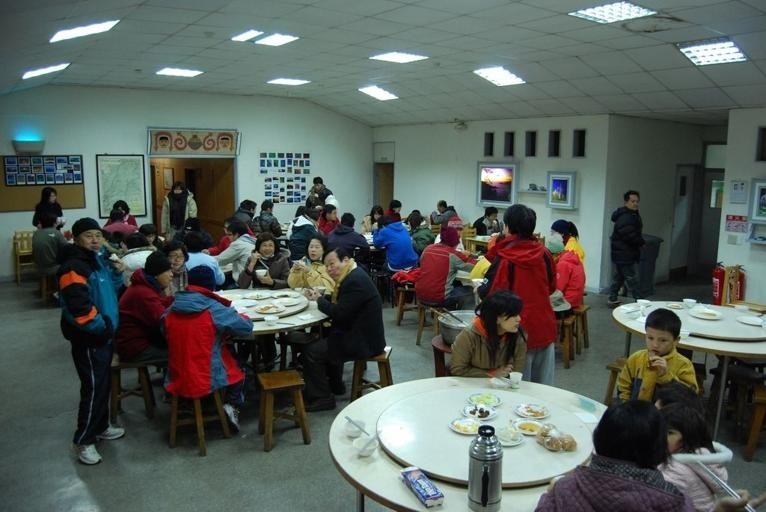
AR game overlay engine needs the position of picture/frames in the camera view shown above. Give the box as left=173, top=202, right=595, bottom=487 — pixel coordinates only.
left=3, top=155, right=83, bottom=186
left=96, top=154, right=147, bottom=219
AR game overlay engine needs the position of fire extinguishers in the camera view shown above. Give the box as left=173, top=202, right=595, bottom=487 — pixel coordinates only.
left=729, top=264, right=746, bottom=303
left=712, top=260, right=726, bottom=305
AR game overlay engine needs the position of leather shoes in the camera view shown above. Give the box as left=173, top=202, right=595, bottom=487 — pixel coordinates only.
left=303, top=394, right=336, bottom=412
left=330, top=381, right=346, bottom=395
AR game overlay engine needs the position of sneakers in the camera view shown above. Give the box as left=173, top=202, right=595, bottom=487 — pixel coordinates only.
left=70, top=443, right=102, bottom=465
left=608, top=299, right=623, bottom=308
left=95, top=426, right=125, bottom=440
left=223, top=403, right=241, bottom=432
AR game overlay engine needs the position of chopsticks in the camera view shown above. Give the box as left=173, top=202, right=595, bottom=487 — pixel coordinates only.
left=258, top=259, right=269, bottom=269
left=696, top=460, right=757, bottom=512
left=345, top=416, right=370, bottom=436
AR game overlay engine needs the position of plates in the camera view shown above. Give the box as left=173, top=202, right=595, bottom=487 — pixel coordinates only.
left=217, top=289, right=301, bottom=314
left=448, top=392, right=552, bottom=447
left=666, top=302, right=764, bottom=326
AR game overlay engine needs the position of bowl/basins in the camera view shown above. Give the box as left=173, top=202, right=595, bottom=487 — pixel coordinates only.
left=686, top=300, right=697, bottom=308
left=255, top=269, right=267, bottom=277
left=353, top=437, right=379, bottom=457
left=344, top=420, right=366, bottom=437
left=313, top=287, right=326, bottom=295
left=637, top=299, right=650, bottom=306
left=683, top=299, right=695, bottom=305
left=492, top=379, right=509, bottom=388
left=264, top=315, right=279, bottom=326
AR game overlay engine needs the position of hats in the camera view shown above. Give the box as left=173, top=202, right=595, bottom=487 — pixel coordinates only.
left=145, top=251, right=173, bottom=275
left=71, top=217, right=102, bottom=238
left=184, top=217, right=201, bottom=230
left=187, top=266, right=217, bottom=291
left=441, top=226, right=459, bottom=246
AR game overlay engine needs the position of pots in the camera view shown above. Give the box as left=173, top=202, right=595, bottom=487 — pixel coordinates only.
left=438, top=309, right=479, bottom=345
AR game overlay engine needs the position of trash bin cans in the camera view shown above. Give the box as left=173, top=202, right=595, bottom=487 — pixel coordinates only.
left=617, top=233, right=665, bottom=298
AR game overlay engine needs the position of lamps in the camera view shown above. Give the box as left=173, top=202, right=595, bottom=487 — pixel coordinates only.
left=12, top=140, right=44, bottom=154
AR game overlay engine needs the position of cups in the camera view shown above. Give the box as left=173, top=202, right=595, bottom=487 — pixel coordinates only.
left=366, top=232, right=371, bottom=239
left=509, top=372, right=522, bottom=389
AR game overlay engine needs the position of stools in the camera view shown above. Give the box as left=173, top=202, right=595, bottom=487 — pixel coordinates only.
left=605, top=356, right=628, bottom=406
left=726, top=299, right=765, bottom=312
left=743, top=385, right=766, bottom=462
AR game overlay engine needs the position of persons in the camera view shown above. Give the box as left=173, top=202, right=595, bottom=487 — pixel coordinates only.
left=31, top=212, right=69, bottom=300
left=606, top=189, right=645, bottom=308
left=360, top=198, right=586, bottom=389
left=103, top=175, right=388, bottom=435
left=532, top=378, right=766, bottom=512
left=32, top=186, right=63, bottom=230
left=616, top=307, right=700, bottom=407
left=55, top=216, right=128, bottom=467
